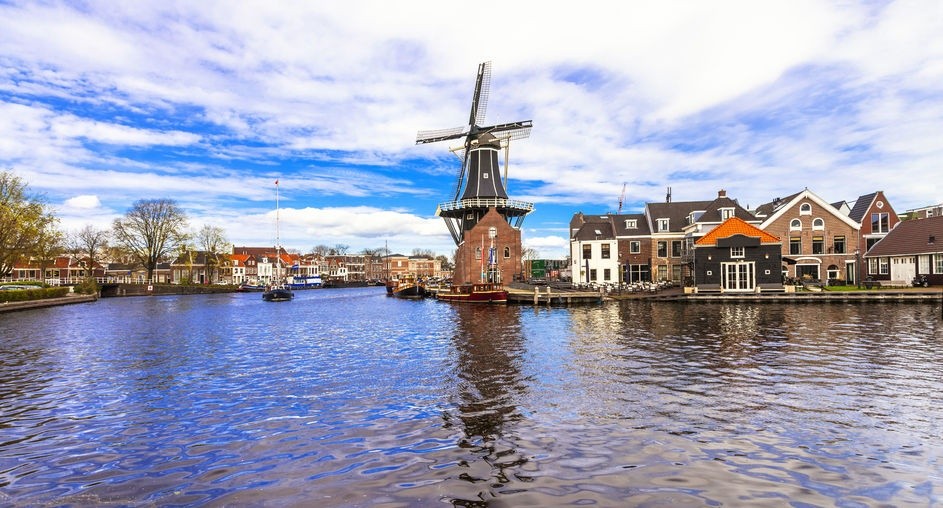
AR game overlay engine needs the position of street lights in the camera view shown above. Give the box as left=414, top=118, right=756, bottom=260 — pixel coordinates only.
left=854, top=246, right=863, bottom=289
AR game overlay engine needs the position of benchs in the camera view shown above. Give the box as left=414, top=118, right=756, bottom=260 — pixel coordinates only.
left=863, top=281, right=881, bottom=289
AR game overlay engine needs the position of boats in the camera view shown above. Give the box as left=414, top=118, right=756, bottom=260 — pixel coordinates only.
left=262, top=177, right=294, bottom=302
left=383, top=278, right=403, bottom=296
left=241, top=280, right=267, bottom=291
left=438, top=232, right=511, bottom=305
left=392, top=281, right=428, bottom=300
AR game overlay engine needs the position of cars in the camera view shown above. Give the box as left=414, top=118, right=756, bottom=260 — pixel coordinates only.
left=0, top=284, right=39, bottom=291
